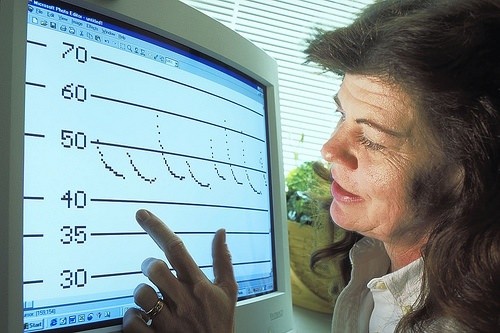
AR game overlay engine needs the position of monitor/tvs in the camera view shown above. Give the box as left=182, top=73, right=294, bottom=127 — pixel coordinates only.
left=0, top=0, right=293, bottom=333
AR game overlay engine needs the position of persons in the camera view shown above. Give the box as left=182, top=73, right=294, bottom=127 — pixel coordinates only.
left=123, top=0, right=500, bottom=333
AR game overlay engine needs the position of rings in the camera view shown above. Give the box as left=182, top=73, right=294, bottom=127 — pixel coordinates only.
left=146, top=298, right=164, bottom=318
